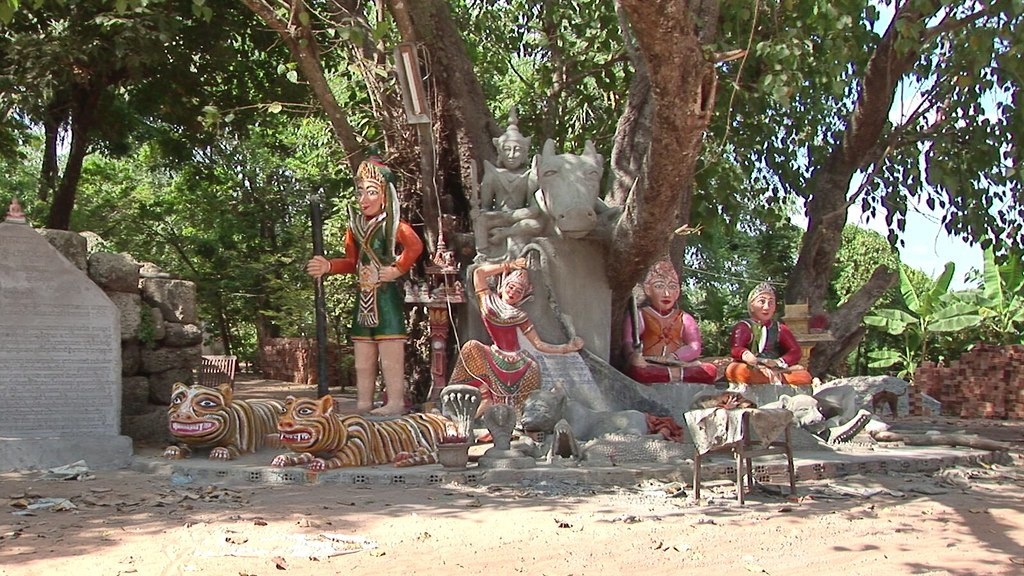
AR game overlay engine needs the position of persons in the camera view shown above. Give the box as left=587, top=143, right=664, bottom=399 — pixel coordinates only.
left=450, top=257, right=583, bottom=431
left=470, top=106, right=548, bottom=270
left=309, top=158, right=423, bottom=416
left=725, top=281, right=812, bottom=384
left=625, top=259, right=717, bottom=385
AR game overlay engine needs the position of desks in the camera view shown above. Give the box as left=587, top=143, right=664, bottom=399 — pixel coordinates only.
left=685, top=406, right=796, bottom=505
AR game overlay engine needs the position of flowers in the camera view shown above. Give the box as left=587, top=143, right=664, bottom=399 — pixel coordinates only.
left=438, top=435, right=465, bottom=442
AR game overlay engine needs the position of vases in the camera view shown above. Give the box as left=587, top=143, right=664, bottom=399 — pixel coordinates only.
left=435, top=444, right=471, bottom=472
